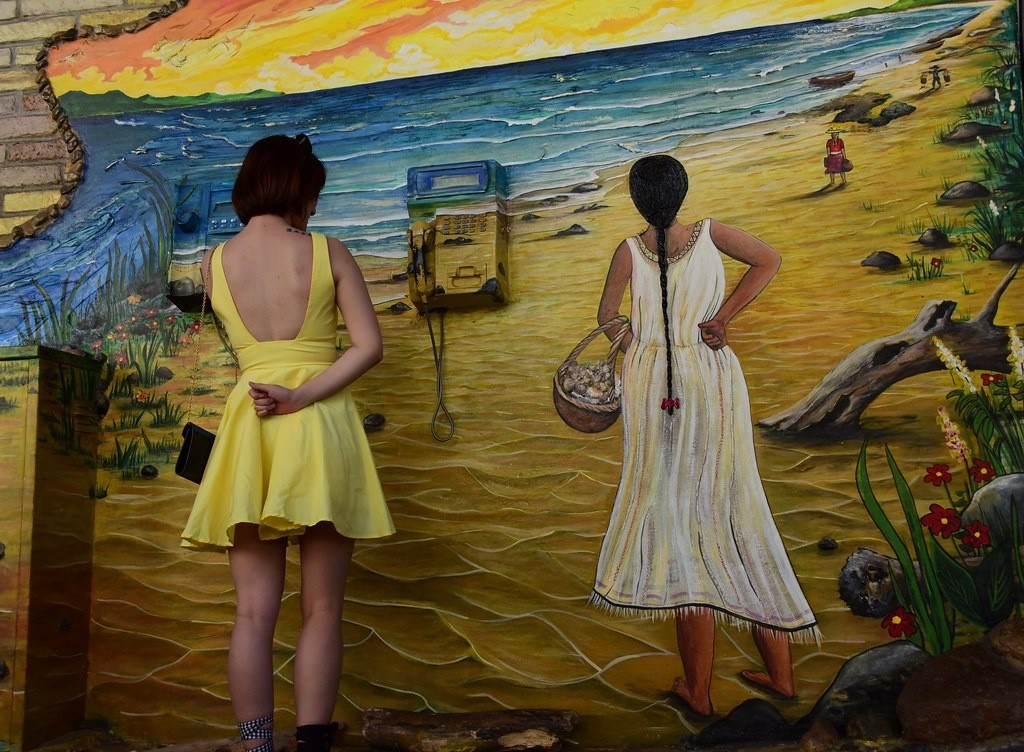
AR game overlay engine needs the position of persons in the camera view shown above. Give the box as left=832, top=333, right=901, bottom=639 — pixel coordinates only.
left=179, top=134, right=396, bottom=752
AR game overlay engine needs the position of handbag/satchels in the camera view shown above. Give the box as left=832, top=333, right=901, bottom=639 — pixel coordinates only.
left=175, top=422, right=216, bottom=485
